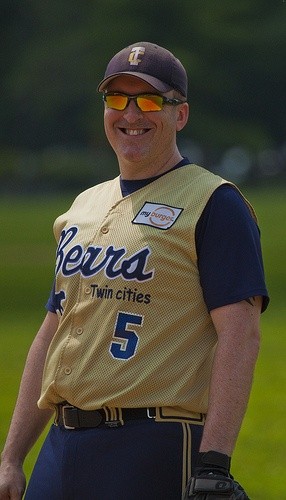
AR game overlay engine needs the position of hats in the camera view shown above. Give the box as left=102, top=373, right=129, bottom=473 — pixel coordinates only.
left=97, top=40, right=189, bottom=99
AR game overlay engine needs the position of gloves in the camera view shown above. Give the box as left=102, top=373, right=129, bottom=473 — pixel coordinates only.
left=183, top=450, right=250, bottom=500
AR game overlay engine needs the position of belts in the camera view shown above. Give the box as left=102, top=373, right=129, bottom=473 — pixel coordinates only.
left=55, top=401, right=157, bottom=430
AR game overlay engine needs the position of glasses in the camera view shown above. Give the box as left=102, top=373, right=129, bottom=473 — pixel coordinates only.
left=101, top=92, right=185, bottom=112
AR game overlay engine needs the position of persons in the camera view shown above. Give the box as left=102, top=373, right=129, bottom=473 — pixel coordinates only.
left=0, top=41, right=271, bottom=498
